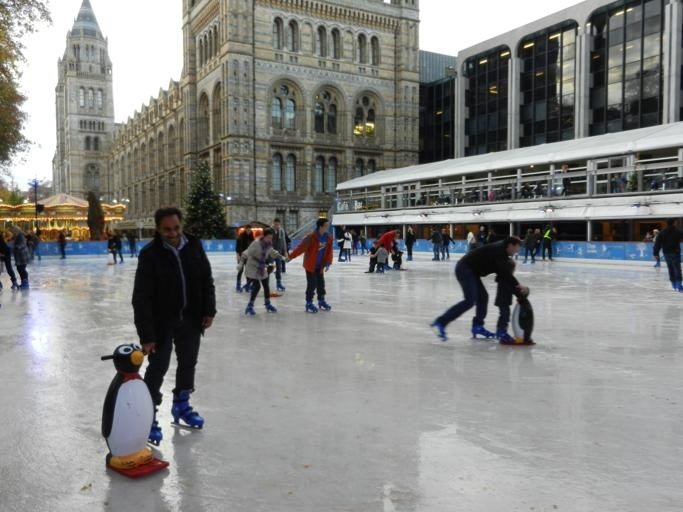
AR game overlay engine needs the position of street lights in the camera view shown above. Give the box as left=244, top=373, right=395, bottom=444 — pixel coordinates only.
left=27, top=178, right=42, bottom=228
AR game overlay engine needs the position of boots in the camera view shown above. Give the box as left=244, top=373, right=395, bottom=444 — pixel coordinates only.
left=428, top=314, right=450, bottom=343
left=244, top=301, right=255, bottom=316
left=305, top=300, right=318, bottom=313
left=676, top=280, right=683, bottom=291
left=169, top=386, right=206, bottom=431
left=242, top=283, right=250, bottom=293
left=11, top=281, right=19, bottom=288
left=653, top=261, right=660, bottom=267
left=148, top=418, right=164, bottom=444
left=235, top=281, right=242, bottom=292
left=472, top=316, right=496, bottom=339
left=317, top=298, right=332, bottom=310
left=670, top=281, right=676, bottom=289
left=20, top=279, right=29, bottom=290
left=496, top=326, right=517, bottom=345
left=264, top=301, right=278, bottom=314
left=276, top=282, right=285, bottom=291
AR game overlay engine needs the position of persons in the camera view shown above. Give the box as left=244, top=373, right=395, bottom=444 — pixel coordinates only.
left=132, top=208, right=216, bottom=444
left=524, top=228, right=557, bottom=263
left=456, top=182, right=544, bottom=204
left=442, top=229, right=455, bottom=261
left=107, top=229, right=139, bottom=264
left=653, top=220, right=683, bottom=292
left=561, top=164, right=571, bottom=196
left=431, top=237, right=523, bottom=340
left=495, top=259, right=523, bottom=340
left=338, top=227, right=403, bottom=273
left=467, top=227, right=496, bottom=250
left=613, top=173, right=626, bottom=193
left=405, top=227, right=416, bottom=261
left=237, top=227, right=287, bottom=316
left=287, top=217, right=333, bottom=312
left=428, top=228, right=442, bottom=261
left=236, top=218, right=291, bottom=293
left=0, top=225, right=40, bottom=289
left=58, top=231, right=66, bottom=259
